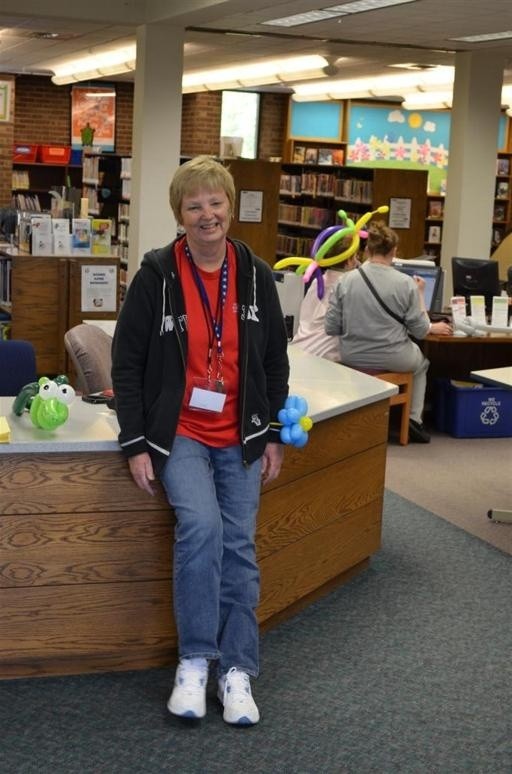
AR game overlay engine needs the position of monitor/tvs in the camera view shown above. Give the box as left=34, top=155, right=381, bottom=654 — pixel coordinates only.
left=451, top=257, right=501, bottom=316
left=390, top=261, right=442, bottom=317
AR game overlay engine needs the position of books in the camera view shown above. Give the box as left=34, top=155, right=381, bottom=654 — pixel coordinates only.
left=117, top=224, right=128, bottom=243
left=123, top=180, right=132, bottom=200
left=0, top=257, right=13, bottom=306
left=118, top=159, right=132, bottom=179
left=118, top=267, right=127, bottom=285
left=277, top=235, right=315, bottom=256
left=337, top=180, right=373, bottom=204
left=429, top=225, right=440, bottom=243
left=278, top=174, right=302, bottom=198
left=496, top=159, right=509, bottom=174
left=82, top=157, right=98, bottom=180
left=293, top=146, right=343, bottom=164
left=278, top=204, right=329, bottom=226
left=11, top=170, right=30, bottom=190
left=428, top=201, right=441, bottom=219
left=116, top=245, right=128, bottom=259
left=13, top=187, right=113, bottom=258
left=118, top=204, right=130, bottom=222
left=335, top=212, right=368, bottom=230
left=495, top=206, right=505, bottom=220
left=496, top=182, right=509, bottom=197
left=302, top=173, right=336, bottom=196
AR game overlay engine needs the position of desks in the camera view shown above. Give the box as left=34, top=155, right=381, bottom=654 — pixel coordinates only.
left=0, top=320, right=399, bottom=681
left=409, top=311, right=512, bottom=359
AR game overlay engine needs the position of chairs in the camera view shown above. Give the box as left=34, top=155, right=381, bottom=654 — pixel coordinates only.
left=1, top=339, right=37, bottom=396
left=64, top=320, right=118, bottom=396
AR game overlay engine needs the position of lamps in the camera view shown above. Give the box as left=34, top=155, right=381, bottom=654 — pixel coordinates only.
left=48, top=41, right=137, bottom=87
left=258, top=9, right=349, bottom=27
left=446, top=30, right=512, bottom=43
left=182, top=54, right=340, bottom=94
left=399, top=93, right=452, bottom=111
left=501, top=83, right=512, bottom=107
left=280, top=71, right=454, bottom=103
left=322, top=0, right=418, bottom=14
left=386, top=61, right=443, bottom=72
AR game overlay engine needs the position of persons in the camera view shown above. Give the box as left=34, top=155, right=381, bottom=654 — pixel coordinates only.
left=111, top=154, right=289, bottom=725
left=325, top=221, right=430, bottom=441
left=289, top=236, right=454, bottom=363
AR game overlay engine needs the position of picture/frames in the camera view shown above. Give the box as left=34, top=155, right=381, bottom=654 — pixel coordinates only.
left=70, top=85, right=117, bottom=155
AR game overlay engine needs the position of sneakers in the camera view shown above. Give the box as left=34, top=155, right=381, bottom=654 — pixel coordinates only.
left=409, top=418, right=430, bottom=442
left=168, top=658, right=208, bottom=719
left=217, top=668, right=260, bottom=726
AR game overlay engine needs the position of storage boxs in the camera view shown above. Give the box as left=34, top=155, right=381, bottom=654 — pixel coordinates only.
left=435, top=374, right=512, bottom=439
left=40, top=142, right=71, bottom=164
left=12, top=143, right=37, bottom=162
left=72, top=145, right=83, bottom=165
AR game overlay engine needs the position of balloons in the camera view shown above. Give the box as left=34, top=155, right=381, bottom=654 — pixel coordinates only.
left=12, top=375, right=75, bottom=433
left=274, top=205, right=391, bottom=299
left=278, top=396, right=314, bottom=448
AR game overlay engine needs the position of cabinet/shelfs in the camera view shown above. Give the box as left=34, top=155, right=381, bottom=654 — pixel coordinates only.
left=489, top=152, right=511, bottom=262
left=10, top=165, right=83, bottom=218
left=284, top=137, right=348, bottom=166
left=426, top=196, right=444, bottom=266
left=113, top=154, right=131, bottom=300
left=82, top=154, right=100, bottom=220
left=0, top=239, right=120, bottom=377
left=276, top=163, right=429, bottom=271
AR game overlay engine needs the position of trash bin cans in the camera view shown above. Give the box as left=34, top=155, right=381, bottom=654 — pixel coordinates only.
left=450, top=379, right=512, bottom=438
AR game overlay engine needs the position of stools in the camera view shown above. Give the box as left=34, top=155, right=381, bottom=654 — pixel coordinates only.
left=424, top=381, right=446, bottom=434
left=340, top=359, right=413, bottom=446
